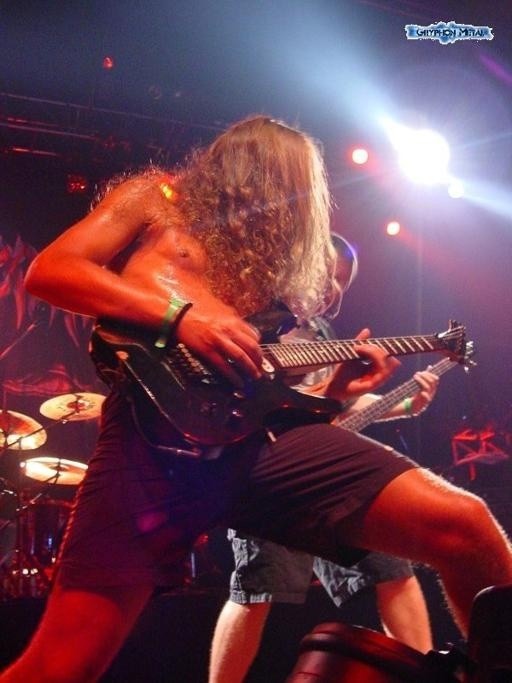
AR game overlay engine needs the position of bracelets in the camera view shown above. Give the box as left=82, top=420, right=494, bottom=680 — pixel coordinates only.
left=153, top=297, right=191, bottom=350
left=405, top=396, right=414, bottom=417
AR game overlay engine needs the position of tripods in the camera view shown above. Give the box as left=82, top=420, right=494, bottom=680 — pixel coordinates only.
left=0, top=446, right=57, bottom=588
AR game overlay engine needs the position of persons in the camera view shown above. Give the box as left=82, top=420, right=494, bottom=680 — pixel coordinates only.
left=206, top=232, right=441, bottom=683
left=0, top=114, right=512, bottom=683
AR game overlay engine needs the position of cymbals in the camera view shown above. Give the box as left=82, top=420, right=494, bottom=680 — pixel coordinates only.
left=39, top=391, right=108, bottom=421
left=1, top=411, right=47, bottom=452
left=22, top=458, right=89, bottom=486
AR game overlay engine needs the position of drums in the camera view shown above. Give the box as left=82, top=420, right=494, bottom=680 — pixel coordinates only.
left=1, top=478, right=19, bottom=576
left=31, top=499, right=74, bottom=567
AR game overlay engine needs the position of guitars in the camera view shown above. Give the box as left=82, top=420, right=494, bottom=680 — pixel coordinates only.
left=89, top=299, right=467, bottom=460
left=302, top=337, right=478, bottom=434
